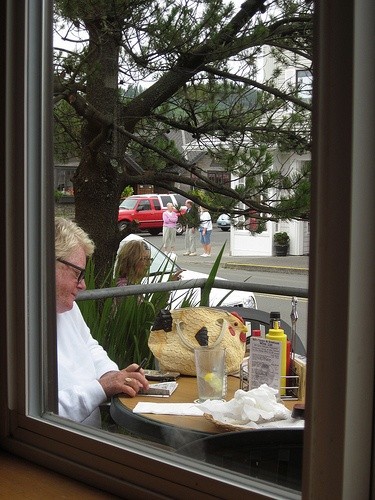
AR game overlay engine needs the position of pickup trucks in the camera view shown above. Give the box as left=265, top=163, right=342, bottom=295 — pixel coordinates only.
left=118, top=197, right=187, bottom=235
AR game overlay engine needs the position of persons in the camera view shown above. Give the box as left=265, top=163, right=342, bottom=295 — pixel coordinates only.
left=163, top=203, right=178, bottom=254
left=54, top=216, right=150, bottom=431
left=183, top=200, right=212, bottom=257
left=106, top=239, right=183, bottom=329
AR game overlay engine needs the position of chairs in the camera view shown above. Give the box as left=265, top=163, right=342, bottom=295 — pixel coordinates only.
left=179, top=427, right=304, bottom=490
left=215, top=307, right=305, bottom=356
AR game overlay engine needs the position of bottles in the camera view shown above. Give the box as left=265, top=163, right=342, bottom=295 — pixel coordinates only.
left=252, top=312, right=290, bottom=397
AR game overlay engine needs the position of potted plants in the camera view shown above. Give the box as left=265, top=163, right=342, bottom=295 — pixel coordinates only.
left=274, top=232, right=290, bottom=256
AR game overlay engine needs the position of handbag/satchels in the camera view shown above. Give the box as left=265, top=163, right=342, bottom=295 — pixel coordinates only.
left=147, top=307, right=246, bottom=375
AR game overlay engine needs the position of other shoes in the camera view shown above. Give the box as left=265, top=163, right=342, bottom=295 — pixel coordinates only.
left=189, top=253, right=197, bottom=256
left=182, top=251, right=191, bottom=255
left=200, top=253, right=211, bottom=258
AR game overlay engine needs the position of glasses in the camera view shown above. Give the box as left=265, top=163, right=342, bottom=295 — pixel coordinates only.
left=56, top=258, right=85, bottom=282
left=139, top=258, right=154, bottom=264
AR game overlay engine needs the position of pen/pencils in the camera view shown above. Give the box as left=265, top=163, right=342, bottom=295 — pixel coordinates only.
left=134, top=357, right=149, bottom=372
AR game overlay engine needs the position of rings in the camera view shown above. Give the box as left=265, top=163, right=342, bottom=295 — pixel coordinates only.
left=124, top=377, right=132, bottom=385
left=174, top=272, right=180, bottom=280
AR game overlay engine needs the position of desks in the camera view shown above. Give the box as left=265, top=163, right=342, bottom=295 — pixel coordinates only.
left=110, top=372, right=306, bottom=448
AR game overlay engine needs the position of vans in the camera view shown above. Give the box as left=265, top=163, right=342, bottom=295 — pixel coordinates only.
left=130, top=193, right=202, bottom=232
left=216, top=214, right=247, bottom=231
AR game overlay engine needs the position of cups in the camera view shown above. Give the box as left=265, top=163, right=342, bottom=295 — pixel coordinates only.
left=194, top=345, right=229, bottom=403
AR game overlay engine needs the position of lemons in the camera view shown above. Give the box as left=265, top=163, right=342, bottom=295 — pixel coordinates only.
left=205, top=372, right=222, bottom=392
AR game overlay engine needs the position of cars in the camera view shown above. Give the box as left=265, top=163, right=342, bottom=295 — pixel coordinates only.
left=112, top=233, right=258, bottom=319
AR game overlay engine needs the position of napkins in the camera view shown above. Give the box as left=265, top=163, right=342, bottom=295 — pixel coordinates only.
left=133, top=402, right=206, bottom=416
